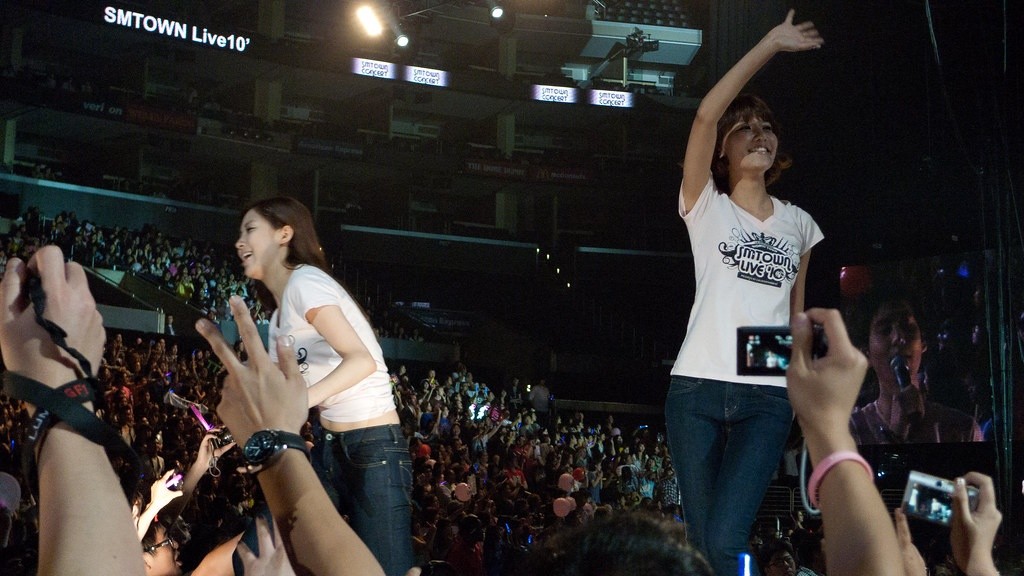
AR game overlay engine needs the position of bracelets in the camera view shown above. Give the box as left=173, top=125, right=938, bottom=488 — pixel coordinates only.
left=807, top=450, right=873, bottom=508
left=874, top=423, right=907, bottom=444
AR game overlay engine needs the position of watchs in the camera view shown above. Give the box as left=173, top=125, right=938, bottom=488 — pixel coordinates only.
left=242, top=430, right=311, bottom=475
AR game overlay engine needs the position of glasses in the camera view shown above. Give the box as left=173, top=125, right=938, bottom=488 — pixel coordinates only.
left=768, top=556, right=795, bottom=565
left=144, top=534, right=175, bottom=553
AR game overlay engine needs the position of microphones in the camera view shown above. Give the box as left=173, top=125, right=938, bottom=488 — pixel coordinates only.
left=888, top=354, right=923, bottom=422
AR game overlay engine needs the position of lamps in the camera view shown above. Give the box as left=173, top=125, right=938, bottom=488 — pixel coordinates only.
left=485, top=0, right=504, bottom=19
left=390, top=18, right=409, bottom=48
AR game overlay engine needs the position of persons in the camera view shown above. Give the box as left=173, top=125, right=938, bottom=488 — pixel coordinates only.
left=910, top=483, right=919, bottom=512
left=234, top=198, right=415, bottom=575
left=931, top=498, right=943, bottom=520
left=766, top=350, right=780, bottom=367
left=1, top=209, right=1024, bottom=576
left=664, top=8, right=826, bottom=576
left=12, top=159, right=56, bottom=182
left=849, top=287, right=985, bottom=446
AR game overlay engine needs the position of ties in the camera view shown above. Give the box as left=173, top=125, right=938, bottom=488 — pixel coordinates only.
left=480, top=439, right=484, bottom=450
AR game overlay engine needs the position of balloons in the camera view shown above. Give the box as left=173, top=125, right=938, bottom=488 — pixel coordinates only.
left=572, top=468, right=585, bottom=481
left=553, top=498, right=571, bottom=517
left=559, top=473, right=575, bottom=491
left=565, top=496, right=576, bottom=510
left=455, top=483, right=471, bottom=501
left=611, top=428, right=620, bottom=436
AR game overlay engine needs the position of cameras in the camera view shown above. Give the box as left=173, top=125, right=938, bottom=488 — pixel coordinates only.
left=213, top=427, right=234, bottom=449
left=900, top=470, right=982, bottom=528
left=162, top=459, right=186, bottom=492
left=736, top=325, right=825, bottom=376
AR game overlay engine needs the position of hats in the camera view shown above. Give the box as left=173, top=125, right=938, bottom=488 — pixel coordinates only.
left=460, top=514, right=478, bottom=530
left=501, top=419, right=512, bottom=427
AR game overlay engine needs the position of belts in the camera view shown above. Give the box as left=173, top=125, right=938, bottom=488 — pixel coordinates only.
left=330, top=427, right=405, bottom=442
